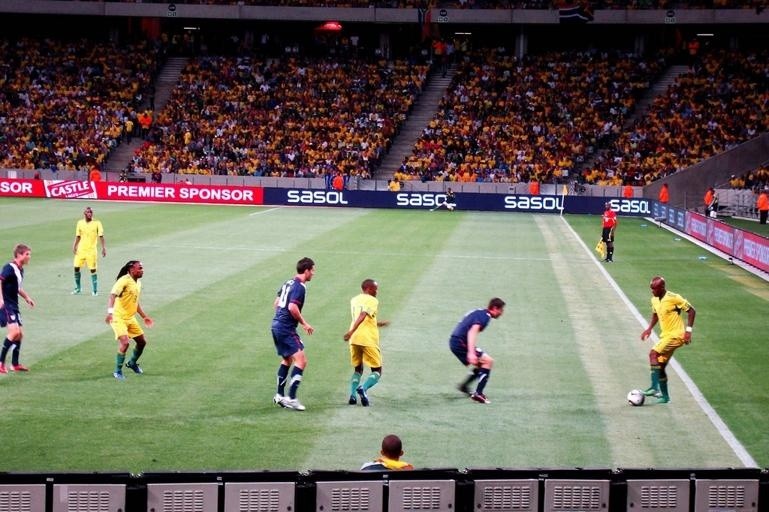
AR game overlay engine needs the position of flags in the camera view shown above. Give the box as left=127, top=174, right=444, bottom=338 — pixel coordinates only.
left=595, top=240, right=605, bottom=259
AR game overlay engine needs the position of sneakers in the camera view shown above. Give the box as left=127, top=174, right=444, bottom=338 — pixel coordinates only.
left=0, top=360, right=11, bottom=375
left=282, top=395, right=306, bottom=411
left=7, top=361, right=29, bottom=372
left=272, top=393, right=286, bottom=408
left=658, top=394, right=670, bottom=405
left=348, top=395, right=358, bottom=405
left=356, top=385, right=370, bottom=407
left=111, top=368, right=126, bottom=381
left=470, top=390, right=492, bottom=403
left=125, top=361, right=143, bottom=374
left=600, top=259, right=614, bottom=264
left=638, top=386, right=661, bottom=396
left=70, top=287, right=82, bottom=296
left=457, top=382, right=476, bottom=396
left=91, top=291, right=98, bottom=297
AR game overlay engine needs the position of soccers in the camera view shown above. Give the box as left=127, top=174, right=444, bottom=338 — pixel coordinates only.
left=627, top=390, right=644, bottom=406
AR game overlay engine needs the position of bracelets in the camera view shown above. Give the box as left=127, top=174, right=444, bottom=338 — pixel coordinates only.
left=107, top=307, right=113, bottom=314
left=685, top=326, right=692, bottom=332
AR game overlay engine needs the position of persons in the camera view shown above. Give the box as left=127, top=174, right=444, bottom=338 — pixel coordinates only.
left=119, top=37, right=468, bottom=192
left=360, top=434, right=412, bottom=470
left=71, top=206, right=106, bottom=297
left=270, top=256, right=315, bottom=411
left=463, top=37, right=769, bottom=227
left=104, top=260, right=153, bottom=380
left=343, top=279, right=389, bottom=407
left=449, top=296, right=506, bottom=405
left=0, top=30, right=177, bottom=179
left=639, top=277, right=696, bottom=404
left=0, top=243, right=34, bottom=374
left=601, top=202, right=617, bottom=264
